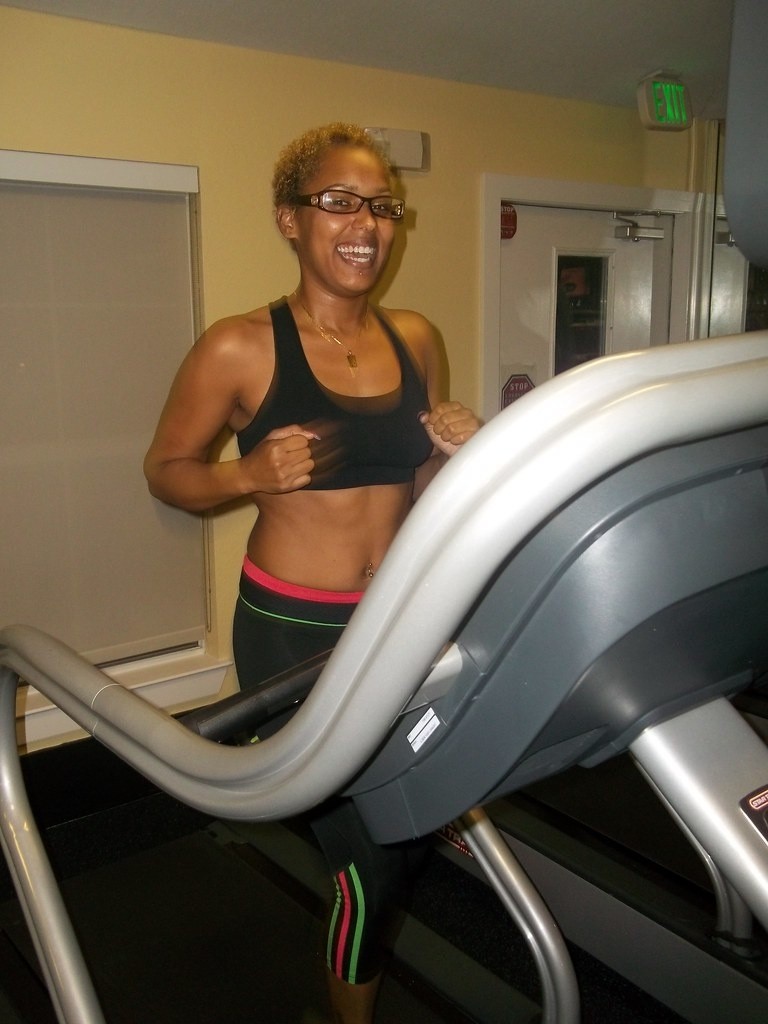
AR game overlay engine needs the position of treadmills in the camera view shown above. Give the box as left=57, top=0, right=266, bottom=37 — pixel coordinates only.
left=0, top=330, right=768, bottom=1024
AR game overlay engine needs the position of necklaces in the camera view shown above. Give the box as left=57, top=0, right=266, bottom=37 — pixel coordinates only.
left=292, top=289, right=370, bottom=370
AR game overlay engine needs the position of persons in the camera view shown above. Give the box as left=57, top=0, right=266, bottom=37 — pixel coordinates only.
left=143, top=124, right=486, bottom=1023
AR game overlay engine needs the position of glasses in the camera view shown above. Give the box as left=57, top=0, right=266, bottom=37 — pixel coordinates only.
left=289, top=190, right=405, bottom=220
